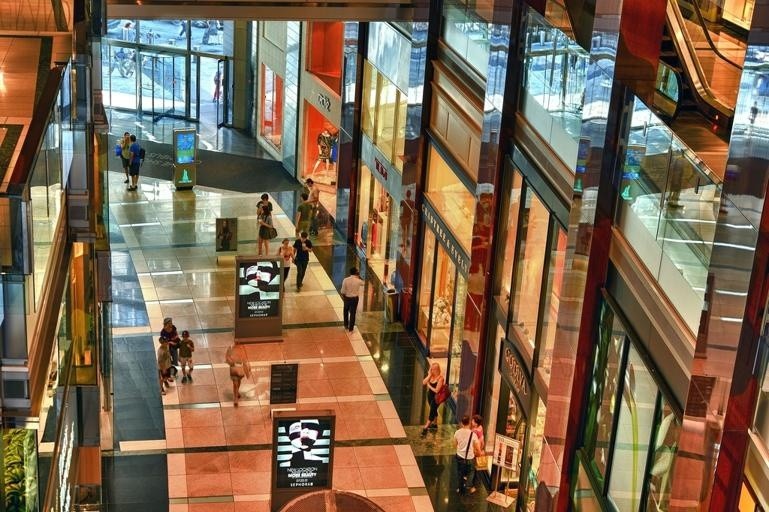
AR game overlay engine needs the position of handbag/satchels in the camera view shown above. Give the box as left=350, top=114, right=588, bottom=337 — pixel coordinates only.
left=464, top=461, right=475, bottom=475
left=474, top=454, right=488, bottom=472
left=258, top=225, right=276, bottom=240
left=434, top=384, right=450, bottom=406
left=229, top=363, right=244, bottom=377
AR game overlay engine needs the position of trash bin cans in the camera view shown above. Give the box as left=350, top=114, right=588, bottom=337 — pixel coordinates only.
left=382, top=288, right=400, bottom=323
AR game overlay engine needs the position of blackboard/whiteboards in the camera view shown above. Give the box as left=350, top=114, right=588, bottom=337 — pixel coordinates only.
left=270, top=363, right=299, bottom=404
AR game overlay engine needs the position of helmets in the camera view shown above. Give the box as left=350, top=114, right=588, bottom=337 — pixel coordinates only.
left=158, top=336, right=168, bottom=342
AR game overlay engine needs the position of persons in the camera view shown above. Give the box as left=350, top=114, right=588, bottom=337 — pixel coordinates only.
left=398, top=186, right=417, bottom=254
left=258, top=206, right=273, bottom=255
left=217, top=219, right=232, bottom=249
left=120, top=132, right=132, bottom=184
left=312, top=130, right=332, bottom=178
left=420, top=363, right=444, bottom=436
left=127, top=134, right=141, bottom=191
left=206, top=20, right=220, bottom=35
left=292, top=231, right=313, bottom=292
left=340, top=267, right=365, bottom=333
left=304, top=178, right=320, bottom=236
left=467, top=414, right=485, bottom=494
left=161, top=318, right=181, bottom=382
left=276, top=238, right=293, bottom=292
left=225, top=342, right=250, bottom=408
left=452, top=415, right=481, bottom=497
left=175, top=19, right=188, bottom=39
left=333, top=129, right=340, bottom=176
left=213, top=70, right=222, bottom=102
left=256, top=194, right=273, bottom=252
left=295, top=193, right=312, bottom=239
left=179, top=331, right=194, bottom=383
left=157, top=336, right=173, bottom=396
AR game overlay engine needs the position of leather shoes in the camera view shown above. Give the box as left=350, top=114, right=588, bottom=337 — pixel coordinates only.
left=161, top=374, right=192, bottom=395
left=421, top=428, right=427, bottom=437
left=124, top=178, right=137, bottom=191
left=427, top=424, right=437, bottom=430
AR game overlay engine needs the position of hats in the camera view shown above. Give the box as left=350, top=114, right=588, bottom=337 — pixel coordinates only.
left=180, top=331, right=188, bottom=337
left=162, top=318, right=171, bottom=324
